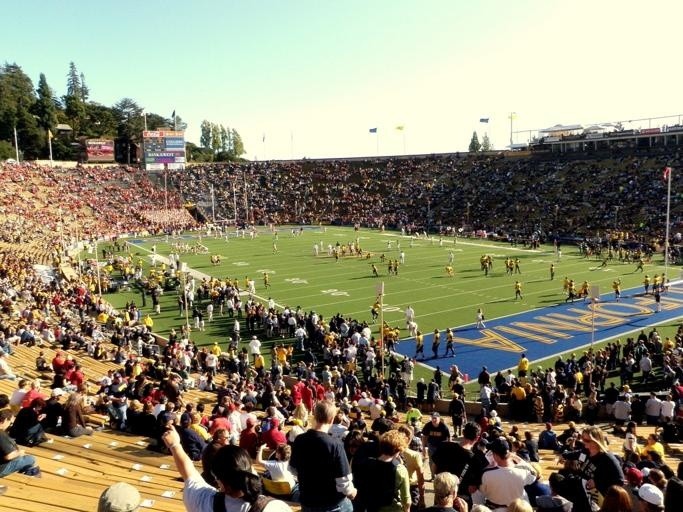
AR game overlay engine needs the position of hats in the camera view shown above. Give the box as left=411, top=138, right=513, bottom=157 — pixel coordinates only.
left=485, top=440, right=508, bottom=450
left=433, top=472, right=459, bottom=496
left=97, top=482, right=140, bottom=512
left=638, top=484, right=664, bottom=509
left=625, top=468, right=643, bottom=484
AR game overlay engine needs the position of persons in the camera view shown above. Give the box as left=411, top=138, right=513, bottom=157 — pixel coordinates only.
left=445, top=256, right=521, bottom=277
left=405, top=306, right=414, bottom=330
left=631, top=260, right=671, bottom=313
left=514, top=281, right=523, bottom=300
left=551, top=264, right=554, bottom=280
left=600, top=259, right=608, bottom=268
left=371, top=259, right=398, bottom=278
left=475, top=309, right=484, bottom=329
left=0, top=145, right=683, bottom=261
left=370, top=300, right=380, bottom=324
left=1, top=260, right=318, bottom=328
left=0, top=314, right=683, bottom=512
left=561, top=277, right=622, bottom=302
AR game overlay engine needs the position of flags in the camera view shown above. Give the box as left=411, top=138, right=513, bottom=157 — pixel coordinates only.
left=512, top=115, right=519, bottom=121
left=480, top=119, right=488, bottom=123
left=396, top=126, right=403, bottom=131
left=369, top=128, right=376, bottom=133
left=48, top=130, right=53, bottom=139
left=171, top=111, right=175, bottom=118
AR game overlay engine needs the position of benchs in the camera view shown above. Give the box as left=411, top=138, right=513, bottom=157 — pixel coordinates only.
left=0, top=162, right=195, bottom=337
